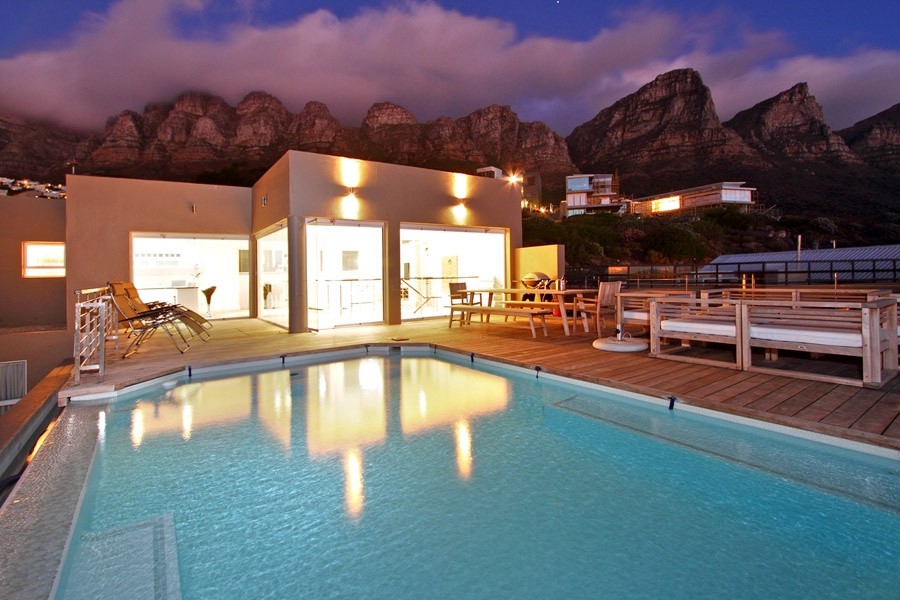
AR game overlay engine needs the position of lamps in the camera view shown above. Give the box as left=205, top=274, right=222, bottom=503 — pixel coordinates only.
left=262, top=196, right=266, bottom=206
left=192, top=205, right=197, bottom=213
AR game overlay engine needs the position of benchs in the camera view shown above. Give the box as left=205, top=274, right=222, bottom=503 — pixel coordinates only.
left=444, top=304, right=552, bottom=338
left=502, top=301, right=605, bottom=331
left=612, top=287, right=900, bottom=389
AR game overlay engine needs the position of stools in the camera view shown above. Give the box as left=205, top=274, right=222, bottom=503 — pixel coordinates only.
left=202, top=286, right=216, bottom=317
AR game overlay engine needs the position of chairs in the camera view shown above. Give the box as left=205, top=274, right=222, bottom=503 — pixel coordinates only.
left=573, top=280, right=622, bottom=338
left=108, top=280, right=213, bottom=358
left=450, top=282, right=484, bottom=325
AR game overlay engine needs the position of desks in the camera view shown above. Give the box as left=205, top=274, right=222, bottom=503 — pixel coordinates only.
left=457, top=288, right=598, bottom=336
